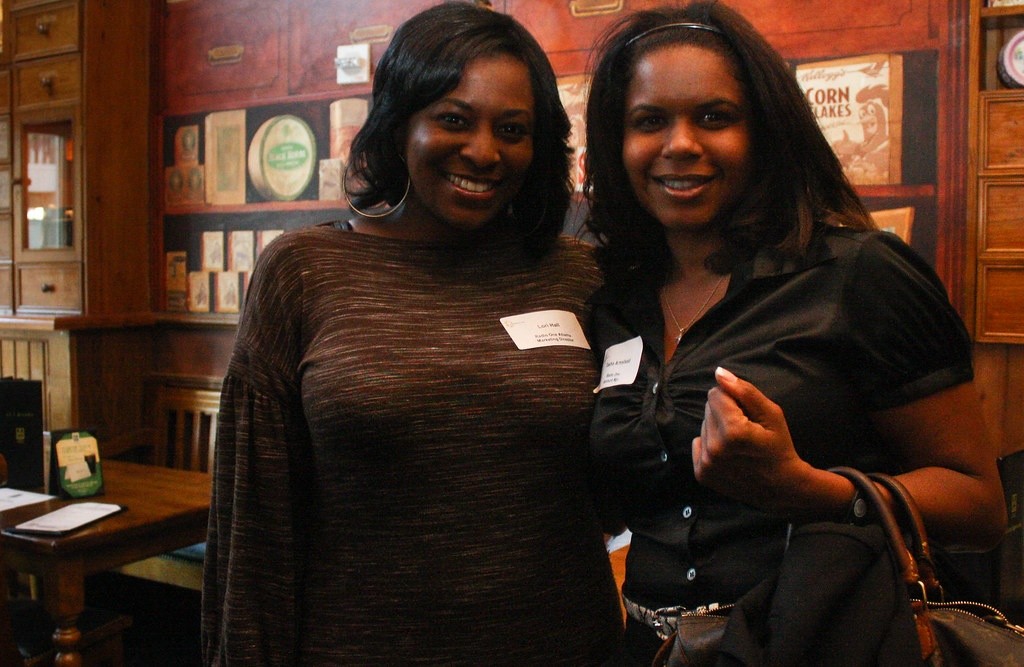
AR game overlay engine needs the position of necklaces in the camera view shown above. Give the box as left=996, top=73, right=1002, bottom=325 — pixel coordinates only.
left=663, top=274, right=724, bottom=345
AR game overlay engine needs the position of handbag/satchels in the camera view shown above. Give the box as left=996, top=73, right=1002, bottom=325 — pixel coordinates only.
left=650, top=463, right=1023, bottom=667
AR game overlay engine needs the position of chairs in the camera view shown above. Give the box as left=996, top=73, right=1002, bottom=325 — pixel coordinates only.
left=1, top=539, right=134, bottom=667
left=108, top=386, right=221, bottom=591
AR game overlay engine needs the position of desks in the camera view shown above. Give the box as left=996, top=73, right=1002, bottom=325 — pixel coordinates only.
left=1, top=459, right=210, bottom=667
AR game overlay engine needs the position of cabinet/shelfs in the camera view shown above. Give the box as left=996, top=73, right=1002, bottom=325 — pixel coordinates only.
left=1, top=1, right=154, bottom=458
left=969, top=0, right=1024, bottom=343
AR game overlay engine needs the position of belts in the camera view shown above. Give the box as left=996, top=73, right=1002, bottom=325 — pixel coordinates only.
left=621, top=594, right=721, bottom=644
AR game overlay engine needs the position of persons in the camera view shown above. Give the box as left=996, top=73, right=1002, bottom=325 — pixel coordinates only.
left=577, top=0, right=1008, bottom=667
left=200, top=2, right=625, bottom=666
